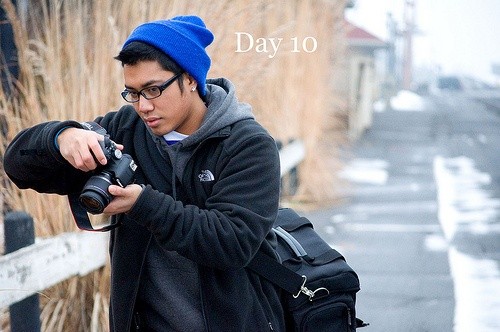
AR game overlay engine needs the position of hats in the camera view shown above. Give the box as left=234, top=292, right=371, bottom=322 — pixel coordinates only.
left=121, top=16, right=214, bottom=96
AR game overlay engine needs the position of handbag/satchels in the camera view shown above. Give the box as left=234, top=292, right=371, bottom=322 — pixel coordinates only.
left=271, top=207, right=370, bottom=332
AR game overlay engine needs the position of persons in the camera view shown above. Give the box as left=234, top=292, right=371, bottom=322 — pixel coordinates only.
left=2, top=13, right=290, bottom=332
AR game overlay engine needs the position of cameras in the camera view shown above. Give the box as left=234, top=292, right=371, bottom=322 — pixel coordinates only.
left=78, top=141, right=141, bottom=216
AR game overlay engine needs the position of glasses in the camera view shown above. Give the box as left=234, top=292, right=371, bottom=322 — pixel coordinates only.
left=122, top=72, right=184, bottom=103
